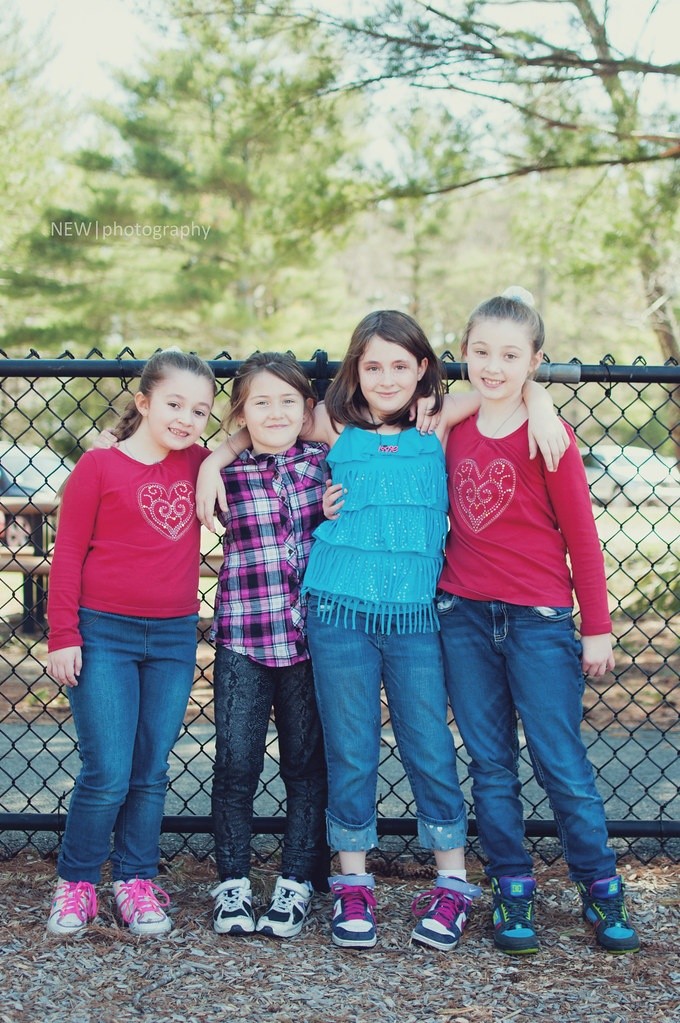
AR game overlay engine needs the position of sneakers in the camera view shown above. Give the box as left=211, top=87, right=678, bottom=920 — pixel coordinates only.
left=47, top=876, right=98, bottom=936
left=112, top=875, right=171, bottom=937
left=256, top=872, right=315, bottom=938
left=490, top=877, right=539, bottom=954
left=211, top=872, right=256, bottom=934
left=576, top=875, right=640, bottom=954
left=411, top=876, right=482, bottom=952
left=327, top=873, right=377, bottom=947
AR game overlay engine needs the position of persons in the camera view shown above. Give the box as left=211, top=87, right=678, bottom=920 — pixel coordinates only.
left=46, top=346, right=213, bottom=935
left=194, top=310, right=571, bottom=952
left=323, top=285, right=639, bottom=954
left=92, top=351, right=440, bottom=938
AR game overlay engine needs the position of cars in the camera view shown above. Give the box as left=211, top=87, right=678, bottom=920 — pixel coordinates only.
left=579, top=443, right=680, bottom=508
left=0, top=442, right=79, bottom=499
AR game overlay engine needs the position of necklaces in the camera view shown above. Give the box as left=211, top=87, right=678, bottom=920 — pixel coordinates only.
left=369, top=410, right=403, bottom=453
left=124, top=439, right=134, bottom=458
left=491, top=401, right=524, bottom=439
